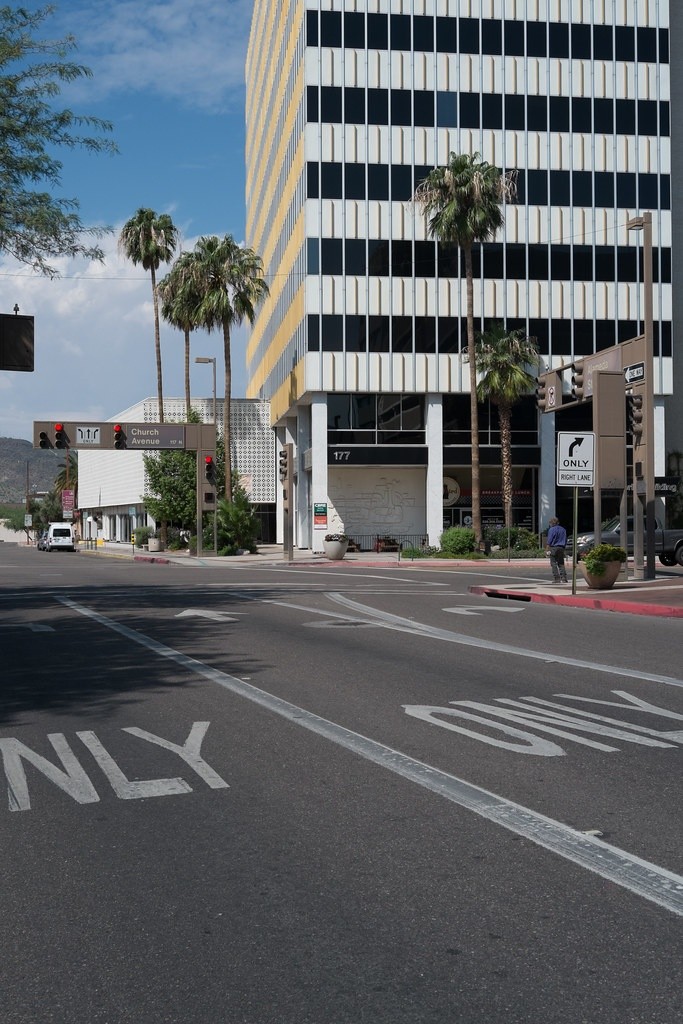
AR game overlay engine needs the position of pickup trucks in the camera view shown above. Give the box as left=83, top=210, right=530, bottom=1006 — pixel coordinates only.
left=565, top=516, right=683, bottom=567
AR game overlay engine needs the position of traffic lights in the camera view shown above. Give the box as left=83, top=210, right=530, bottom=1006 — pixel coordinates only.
left=205, top=456, right=212, bottom=479
left=204, top=492, right=214, bottom=502
left=54, top=423, right=63, bottom=448
left=113, top=425, right=122, bottom=449
left=39, top=431, right=47, bottom=448
left=279, top=450, right=287, bottom=475
left=535, top=376, right=546, bottom=408
left=629, top=395, right=642, bottom=435
left=571, top=363, right=584, bottom=398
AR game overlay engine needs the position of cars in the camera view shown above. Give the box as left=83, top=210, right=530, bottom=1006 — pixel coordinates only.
left=37, top=531, right=48, bottom=550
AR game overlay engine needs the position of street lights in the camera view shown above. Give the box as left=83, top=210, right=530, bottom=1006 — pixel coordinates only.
left=195, top=357, right=216, bottom=425
left=627, top=212, right=652, bottom=335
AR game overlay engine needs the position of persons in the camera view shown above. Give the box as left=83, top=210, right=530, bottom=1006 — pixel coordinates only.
left=178, top=528, right=192, bottom=552
left=545, top=517, right=568, bottom=583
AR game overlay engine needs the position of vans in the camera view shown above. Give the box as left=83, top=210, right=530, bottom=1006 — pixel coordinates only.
left=45, top=522, right=74, bottom=552
